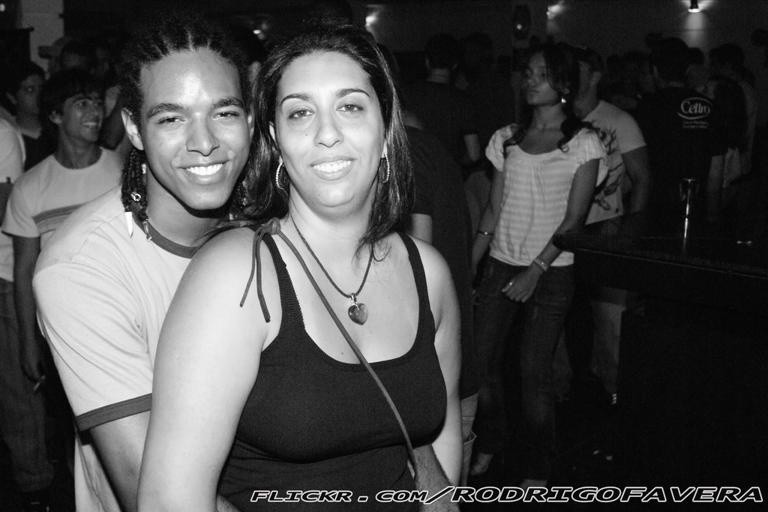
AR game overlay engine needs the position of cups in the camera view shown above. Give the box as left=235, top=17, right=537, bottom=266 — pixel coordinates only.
left=676, top=176, right=697, bottom=203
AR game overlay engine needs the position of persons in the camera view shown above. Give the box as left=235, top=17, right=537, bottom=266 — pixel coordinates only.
left=2, top=0, right=768, bottom=510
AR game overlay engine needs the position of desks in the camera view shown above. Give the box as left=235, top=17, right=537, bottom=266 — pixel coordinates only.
left=550, top=194, right=768, bottom=511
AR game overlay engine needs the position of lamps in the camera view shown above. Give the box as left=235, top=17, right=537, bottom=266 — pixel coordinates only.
left=688, top=0, right=699, bottom=13
